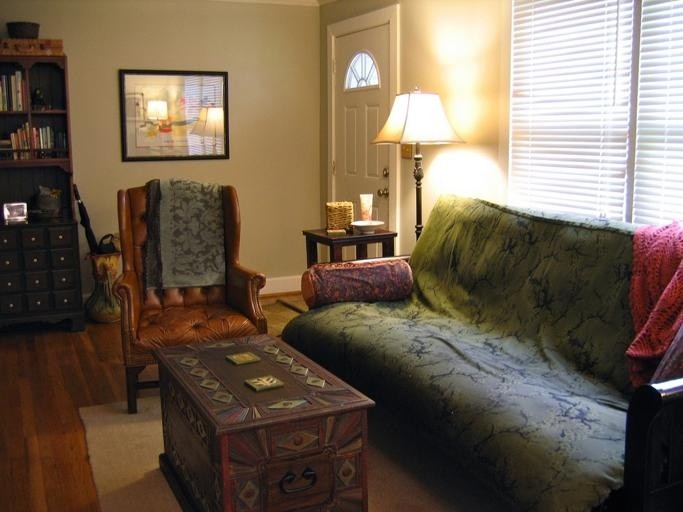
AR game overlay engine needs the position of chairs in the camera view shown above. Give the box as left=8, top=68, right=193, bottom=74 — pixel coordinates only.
left=111, top=184, right=266, bottom=414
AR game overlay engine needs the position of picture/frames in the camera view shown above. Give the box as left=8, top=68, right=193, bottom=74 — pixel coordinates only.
left=3, top=202, right=28, bottom=225
left=118, top=68, right=229, bottom=161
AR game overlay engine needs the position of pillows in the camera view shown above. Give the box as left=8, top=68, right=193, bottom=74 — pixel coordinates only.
left=301, top=257, right=413, bottom=309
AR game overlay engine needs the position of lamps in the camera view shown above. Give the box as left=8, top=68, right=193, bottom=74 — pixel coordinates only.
left=190, top=107, right=224, bottom=153
left=370, top=85, right=466, bottom=242
left=147, top=100, right=166, bottom=120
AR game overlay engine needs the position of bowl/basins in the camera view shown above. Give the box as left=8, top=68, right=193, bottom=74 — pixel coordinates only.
left=350, top=220, right=384, bottom=234
left=6, top=21, right=40, bottom=40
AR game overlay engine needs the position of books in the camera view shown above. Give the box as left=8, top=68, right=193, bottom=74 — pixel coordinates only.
left=0, top=70, right=65, bottom=161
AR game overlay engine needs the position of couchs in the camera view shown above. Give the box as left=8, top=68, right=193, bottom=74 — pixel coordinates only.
left=282, top=190, right=683, bottom=512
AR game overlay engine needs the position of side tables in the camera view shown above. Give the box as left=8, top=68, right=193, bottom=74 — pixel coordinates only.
left=302, top=228, right=398, bottom=268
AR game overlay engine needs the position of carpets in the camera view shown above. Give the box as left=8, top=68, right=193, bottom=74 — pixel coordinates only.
left=261, top=299, right=305, bottom=338
left=80, top=397, right=462, bottom=512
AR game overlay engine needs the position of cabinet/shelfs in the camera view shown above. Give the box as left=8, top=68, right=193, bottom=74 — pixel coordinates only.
left=0, top=55, right=85, bottom=332
